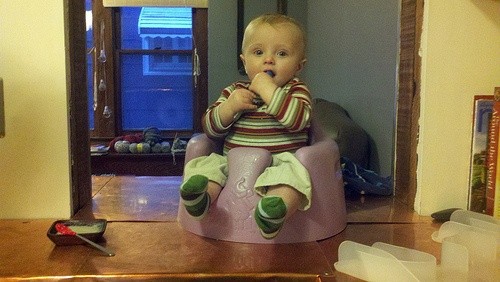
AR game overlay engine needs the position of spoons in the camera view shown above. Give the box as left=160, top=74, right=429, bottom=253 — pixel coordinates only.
left=56, top=223, right=115, bottom=256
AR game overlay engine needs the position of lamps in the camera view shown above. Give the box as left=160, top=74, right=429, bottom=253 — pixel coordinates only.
left=102, top=88, right=111, bottom=119
left=97, top=18, right=107, bottom=63
left=98, top=64, right=106, bottom=91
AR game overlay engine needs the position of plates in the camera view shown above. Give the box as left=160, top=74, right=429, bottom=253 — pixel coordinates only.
left=47, top=219, right=107, bottom=245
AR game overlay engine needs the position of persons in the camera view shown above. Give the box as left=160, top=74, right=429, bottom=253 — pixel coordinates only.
left=181, top=12, right=314, bottom=240
